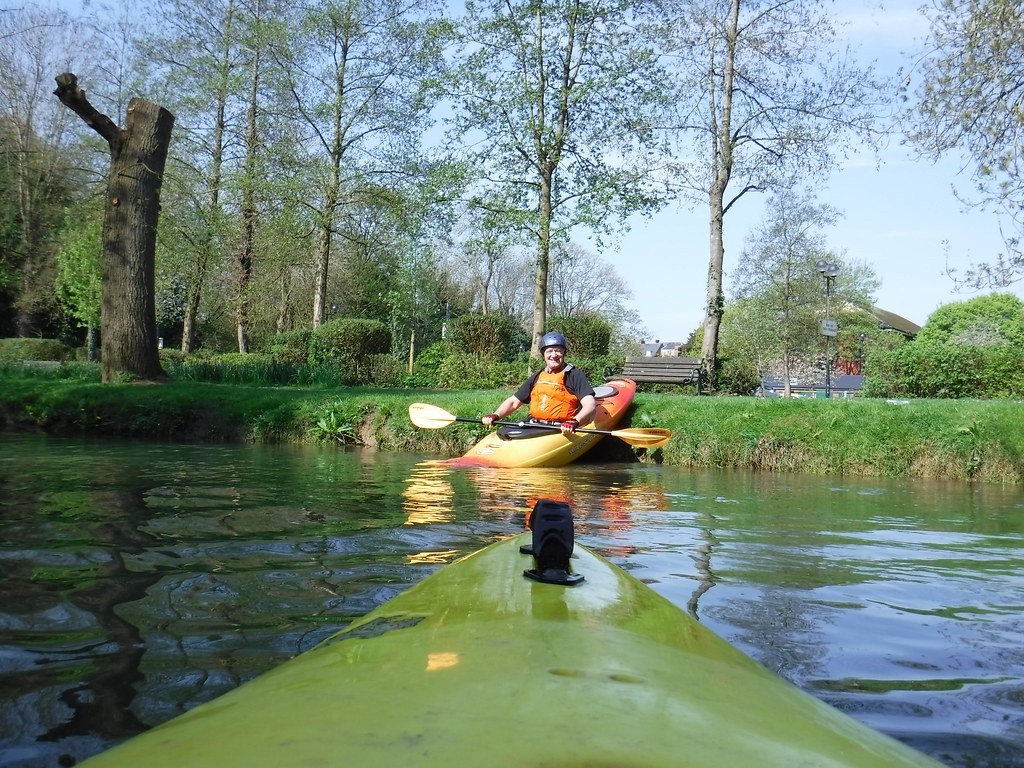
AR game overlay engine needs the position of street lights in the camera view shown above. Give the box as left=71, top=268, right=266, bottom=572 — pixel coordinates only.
left=816, top=259, right=840, bottom=398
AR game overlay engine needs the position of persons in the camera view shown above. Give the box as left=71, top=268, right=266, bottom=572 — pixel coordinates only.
left=482, top=332, right=596, bottom=437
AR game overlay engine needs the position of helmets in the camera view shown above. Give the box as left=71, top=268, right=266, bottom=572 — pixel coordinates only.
left=540, top=331, right=566, bottom=356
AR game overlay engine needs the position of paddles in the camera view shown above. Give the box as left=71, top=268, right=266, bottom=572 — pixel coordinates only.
left=407, top=402, right=672, bottom=449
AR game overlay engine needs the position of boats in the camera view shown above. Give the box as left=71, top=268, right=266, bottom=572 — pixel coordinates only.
left=437, top=373, right=636, bottom=469
